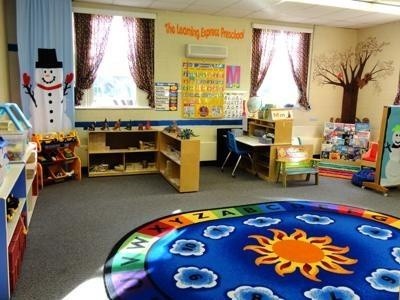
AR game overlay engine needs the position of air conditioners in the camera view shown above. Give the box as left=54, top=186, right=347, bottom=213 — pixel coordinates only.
left=185, top=44, right=229, bottom=59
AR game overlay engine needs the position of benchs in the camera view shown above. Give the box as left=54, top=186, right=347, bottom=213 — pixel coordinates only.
left=275, top=144, right=320, bottom=187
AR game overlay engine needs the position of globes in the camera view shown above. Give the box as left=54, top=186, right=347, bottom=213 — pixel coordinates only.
left=247, top=96, right=262, bottom=118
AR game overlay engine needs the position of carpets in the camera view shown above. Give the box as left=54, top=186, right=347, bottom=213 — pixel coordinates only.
left=103, top=201, right=400, bottom=299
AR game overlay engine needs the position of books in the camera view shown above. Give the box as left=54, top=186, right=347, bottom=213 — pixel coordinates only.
left=320, top=122, right=370, bottom=160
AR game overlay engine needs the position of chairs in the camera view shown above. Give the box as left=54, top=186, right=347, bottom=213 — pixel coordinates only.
left=221, top=130, right=258, bottom=178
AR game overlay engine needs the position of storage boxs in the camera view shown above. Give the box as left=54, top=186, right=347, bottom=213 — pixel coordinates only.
left=0, top=128, right=33, bottom=161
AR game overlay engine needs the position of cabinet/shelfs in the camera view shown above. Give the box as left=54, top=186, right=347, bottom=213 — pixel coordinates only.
left=0, top=136, right=88, bottom=300
left=246, top=116, right=293, bottom=183
left=88, top=131, right=199, bottom=193
